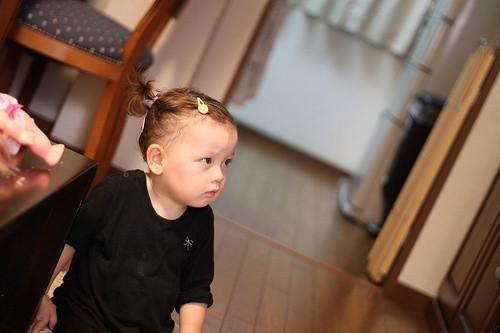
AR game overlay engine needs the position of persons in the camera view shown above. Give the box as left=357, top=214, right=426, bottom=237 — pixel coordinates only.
left=0, top=92, right=64, bottom=180
left=28, top=80, right=238, bottom=333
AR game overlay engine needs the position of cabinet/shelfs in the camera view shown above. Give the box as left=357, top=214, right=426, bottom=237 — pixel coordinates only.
left=429, top=164, right=500, bottom=333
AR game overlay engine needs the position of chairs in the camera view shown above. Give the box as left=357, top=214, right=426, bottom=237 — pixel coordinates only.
left=10, top=0, right=189, bottom=202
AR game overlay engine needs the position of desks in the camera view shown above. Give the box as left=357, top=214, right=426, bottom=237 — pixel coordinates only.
left=0, top=134, right=99, bottom=333
